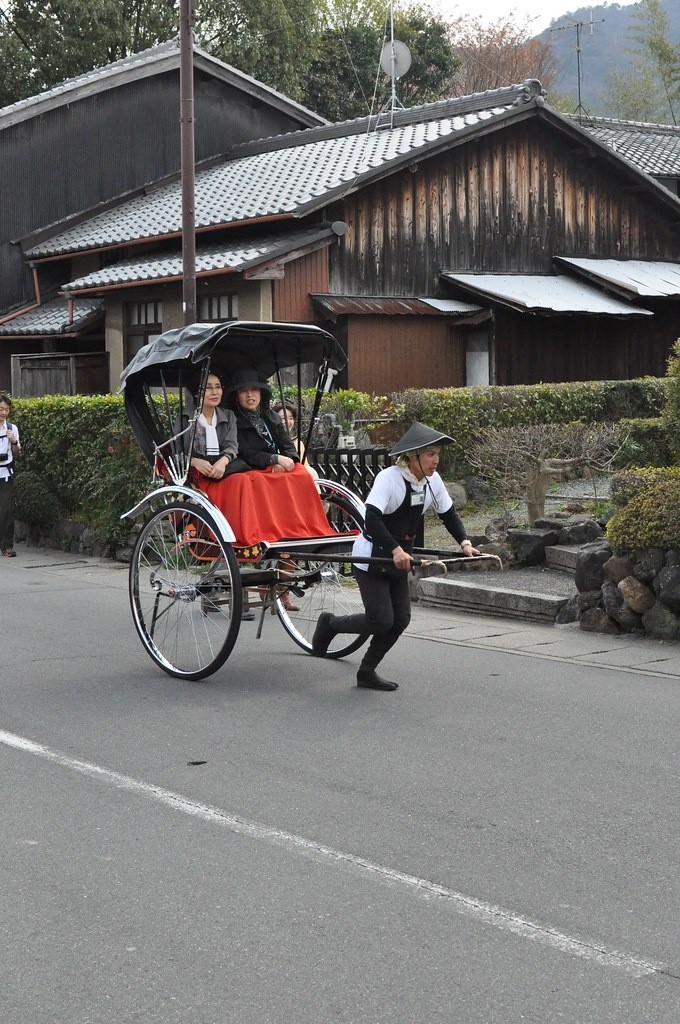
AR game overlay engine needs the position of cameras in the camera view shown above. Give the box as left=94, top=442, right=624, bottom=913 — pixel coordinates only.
left=0, top=453, right=8, bottom=461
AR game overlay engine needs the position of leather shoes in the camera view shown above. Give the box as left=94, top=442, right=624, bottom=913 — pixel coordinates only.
left=312, top=612, right=338, bottom=656
left=357, top=670, right=396, bottom=691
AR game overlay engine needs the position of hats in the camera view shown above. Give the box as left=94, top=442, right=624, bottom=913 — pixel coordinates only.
left=387, top=422, right=456, bottom=456
left=228, top=367, right=272, bottom=401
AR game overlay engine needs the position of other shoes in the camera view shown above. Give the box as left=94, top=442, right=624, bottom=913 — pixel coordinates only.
left=238, top=612, right=254, bottom=620
left=210, top=605, right=221, bottom=612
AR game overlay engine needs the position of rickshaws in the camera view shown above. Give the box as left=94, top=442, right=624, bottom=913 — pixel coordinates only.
left=120, top=319, right=502, bottom=681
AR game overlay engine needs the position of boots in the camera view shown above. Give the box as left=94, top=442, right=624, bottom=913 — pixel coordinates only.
left=279, top=559, right=299, bottom=611
left=260, top=585, right=266, bottom=600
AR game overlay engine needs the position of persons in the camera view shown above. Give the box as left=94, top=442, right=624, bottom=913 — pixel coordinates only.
left=170, top=371, right=327, bottom=620
left=0, top=395, right=21, bottom=557
left=313, top=422, right=481, bottom=691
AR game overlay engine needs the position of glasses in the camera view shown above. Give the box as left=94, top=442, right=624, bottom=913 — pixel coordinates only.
left=206, top=383, right=221, bottom=391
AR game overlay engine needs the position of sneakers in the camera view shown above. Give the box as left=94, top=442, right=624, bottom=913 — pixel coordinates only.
left=2, top=548, right=16, bottom=556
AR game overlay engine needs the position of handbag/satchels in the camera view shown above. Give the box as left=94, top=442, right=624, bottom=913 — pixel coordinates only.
left=198, top=455, right=252, bottom=482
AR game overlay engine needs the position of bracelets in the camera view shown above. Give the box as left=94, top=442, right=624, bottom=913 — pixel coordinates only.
left=11, top=440, right=18, bottom=445
